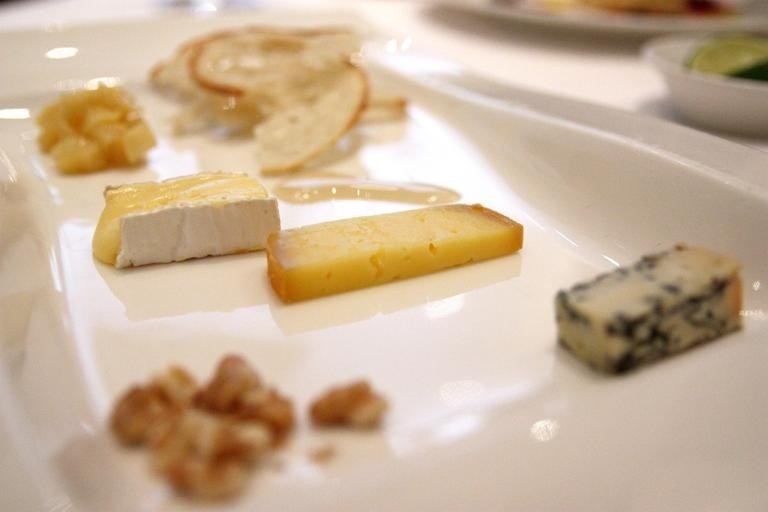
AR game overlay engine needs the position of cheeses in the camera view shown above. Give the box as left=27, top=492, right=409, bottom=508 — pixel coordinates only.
left=266, top=202, right=524, bottom=304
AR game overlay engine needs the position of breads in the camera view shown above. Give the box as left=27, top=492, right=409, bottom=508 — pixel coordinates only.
left=553, top=243, right=744, bottom=371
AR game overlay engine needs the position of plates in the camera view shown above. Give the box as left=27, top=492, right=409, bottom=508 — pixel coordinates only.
left=1, top=6, right=768, bottom=510
